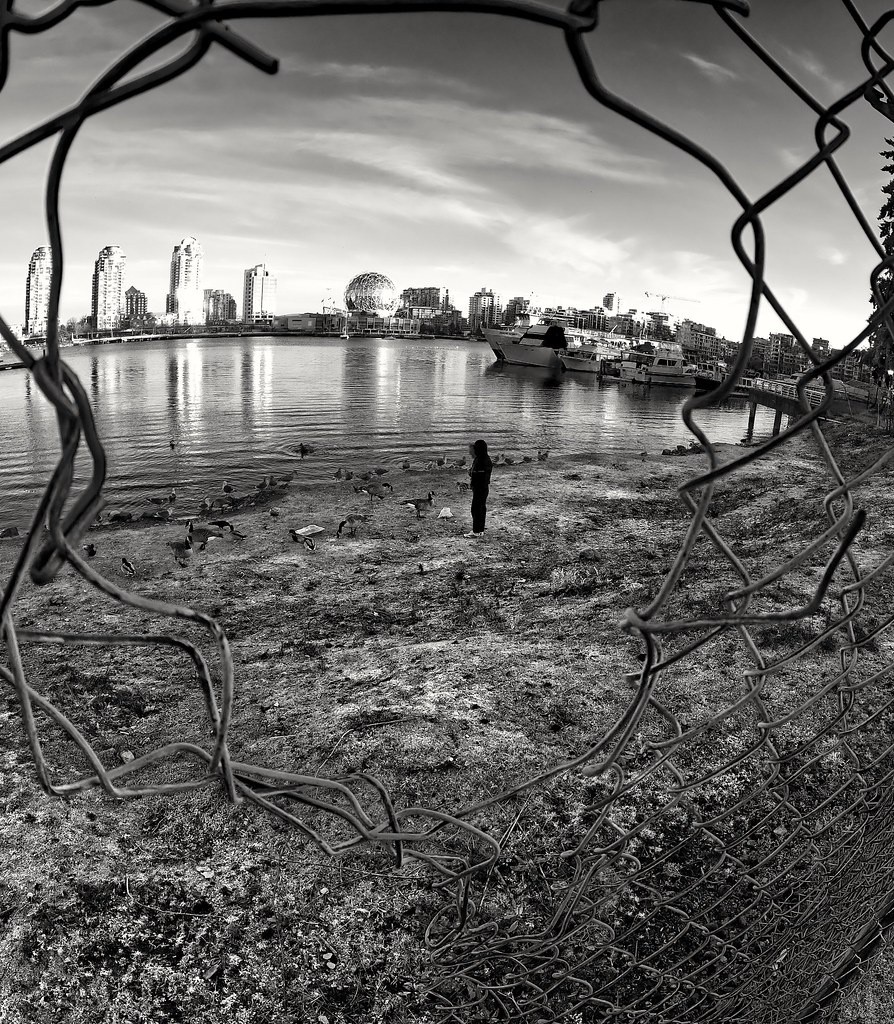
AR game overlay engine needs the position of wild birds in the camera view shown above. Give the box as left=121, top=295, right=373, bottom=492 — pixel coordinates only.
left=84, top=435, right=550, bottom=581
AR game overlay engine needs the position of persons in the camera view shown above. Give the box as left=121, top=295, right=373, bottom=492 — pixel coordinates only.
left=464, top=440, right=493, bottom=537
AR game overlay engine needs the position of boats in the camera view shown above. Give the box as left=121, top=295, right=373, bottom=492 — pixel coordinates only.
left=480, top=311, right=755, bottom=397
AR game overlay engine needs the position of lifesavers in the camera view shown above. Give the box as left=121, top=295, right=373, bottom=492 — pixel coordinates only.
left=708, top=372, right=713, bottom=378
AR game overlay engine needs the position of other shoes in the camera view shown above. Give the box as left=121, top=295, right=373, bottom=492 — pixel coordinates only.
left=464, top=529, right=484, bottom=538
